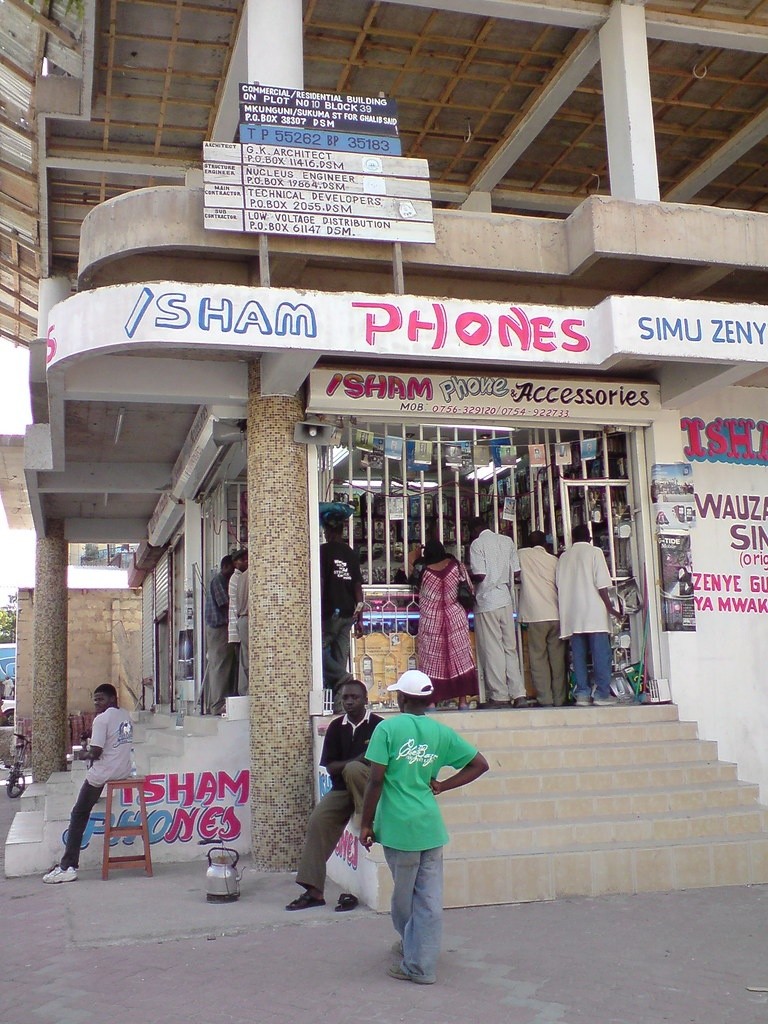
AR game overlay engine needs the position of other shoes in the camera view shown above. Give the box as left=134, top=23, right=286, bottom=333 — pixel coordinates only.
left=594, top=695, right=618, bottom=706
left=513, top=696, right=528, bottom=708
left=478, top=699, right=510, bottom=709
left=391, top=940, right=405, bottom=958
left=332, top=676, right=354, bottom=705
left=459, top=702, right=469, bottom=710
left=576, top=695, right=594, bottom=706
left=425, top=704, right=435, bottom=710
left=388, top=962, right=437, bottom=983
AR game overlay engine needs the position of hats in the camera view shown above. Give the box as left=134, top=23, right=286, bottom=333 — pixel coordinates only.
left=387, top=670, right=433, bottom=696
left=232, top=550, right=246, bottom=560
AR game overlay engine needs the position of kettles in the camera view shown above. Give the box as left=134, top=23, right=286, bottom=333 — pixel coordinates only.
left=205, top=847, right=246, bottom=896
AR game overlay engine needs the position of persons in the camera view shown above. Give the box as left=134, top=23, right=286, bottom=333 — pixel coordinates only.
left=205, top=550, right=248, bottom=715
left=360, top=670, right=489, bottom=984
left=394, top=517, right=623, bottom=711
left=43, top=683, right=133, bottom=884
left=320, top=513, right=365, bottom=715
left=0, top=675, right=15, bottom=726
left=286, top=680, right=385, bottom=911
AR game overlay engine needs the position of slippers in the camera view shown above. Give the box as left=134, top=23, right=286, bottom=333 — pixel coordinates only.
left=335, top=893, right=359, bottom=912
left=285, top=893, right=326, bottom=911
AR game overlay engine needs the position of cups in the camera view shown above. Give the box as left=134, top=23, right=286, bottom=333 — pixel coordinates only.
left=176, top=698, right=194, bottom=726
left=154, top=704, right=170, bottom=723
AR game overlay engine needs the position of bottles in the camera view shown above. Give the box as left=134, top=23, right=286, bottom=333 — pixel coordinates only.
left=130, top=747, right=137, bottom=775
left=332, top=608, right=340, bottom=620
left=177, top=660, right=193, bottom=681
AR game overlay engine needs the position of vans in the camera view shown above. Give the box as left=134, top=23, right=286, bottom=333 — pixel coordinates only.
left=0, top=643, right=16, bottom=726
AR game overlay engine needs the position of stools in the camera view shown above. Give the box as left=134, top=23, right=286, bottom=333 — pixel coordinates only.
left=102, top=777, right=152, bottom=881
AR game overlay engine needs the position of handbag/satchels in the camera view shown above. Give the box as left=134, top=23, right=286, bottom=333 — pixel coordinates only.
left=455, top=559, right=476, bottom=611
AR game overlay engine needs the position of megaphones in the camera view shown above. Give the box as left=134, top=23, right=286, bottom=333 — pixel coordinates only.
left=213, top=420, right=248, bottom=447
left=294, top=416, right=331, bottom=445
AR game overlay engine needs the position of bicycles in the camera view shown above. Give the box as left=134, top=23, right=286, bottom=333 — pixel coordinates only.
left=5, top=733, right=32, bottom=799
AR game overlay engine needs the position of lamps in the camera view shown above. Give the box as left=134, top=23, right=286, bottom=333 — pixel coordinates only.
left=341, top=479, right=438, bottom=488
left=464, top=457, right=522, bottom=481
left=318, top=441, right=355, bottom=470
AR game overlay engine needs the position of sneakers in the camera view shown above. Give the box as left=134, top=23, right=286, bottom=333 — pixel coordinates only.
left=43, top=866, right=78, bottom=883
